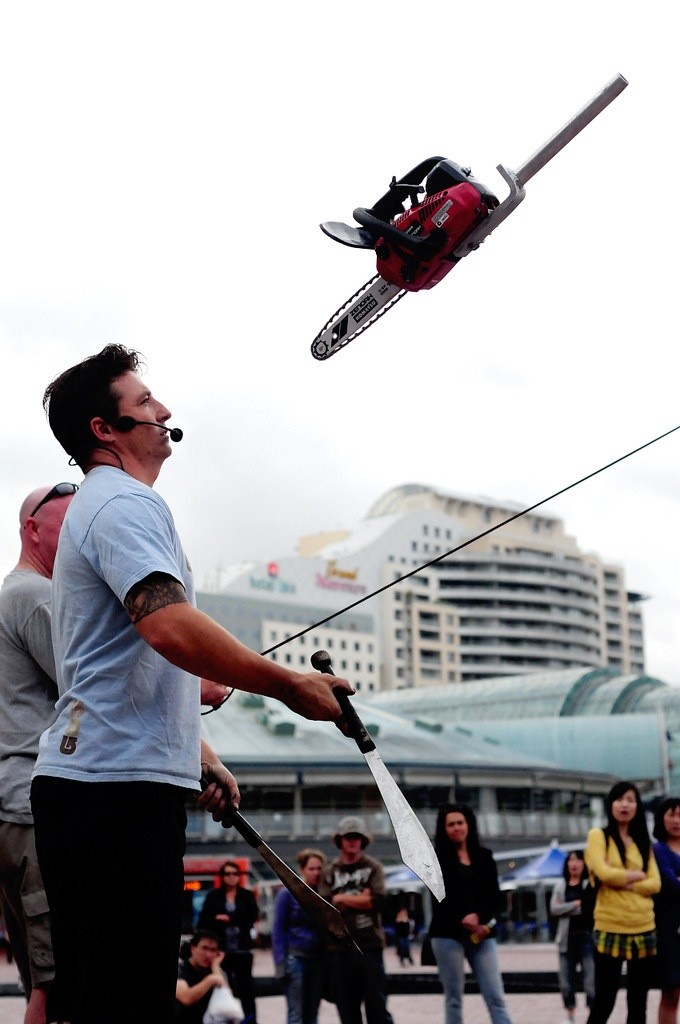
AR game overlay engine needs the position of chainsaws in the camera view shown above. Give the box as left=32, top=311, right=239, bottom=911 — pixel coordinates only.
left=309, top=75, right=630, bottom=362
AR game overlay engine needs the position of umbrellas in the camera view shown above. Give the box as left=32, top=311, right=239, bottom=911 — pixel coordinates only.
left=497, top=842, right=569, bottom=891
left=381, top=865, right=427, bottom=895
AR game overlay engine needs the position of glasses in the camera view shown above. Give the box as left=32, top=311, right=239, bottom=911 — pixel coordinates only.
left=224, top=872, right=240, bottom=876
left=23, top=482, right=79, bottom=530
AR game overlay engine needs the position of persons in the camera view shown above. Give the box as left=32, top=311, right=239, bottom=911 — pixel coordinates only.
left=28, top=341, right=356, bottom=1024
left=316, top=813, right=396, bottom=1024
left=582, top=780, right=660, bottom=1024
left=0, top=484, right=230, bottom=1024
left=193, top=861, right=260, bottom=1024
left=396, top=909, right=414, bottom=965
left=549, top=849, right=596, bottom=1024
left=271, top=848, right=328, bottom=1024
left=174, top=929, right=257, bottom=1024
left=651, top=795, right=680, bottom=1024
left=427, top=802, right=513, bottom=1024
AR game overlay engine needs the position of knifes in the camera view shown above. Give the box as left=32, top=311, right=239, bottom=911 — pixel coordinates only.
left=312, top=651, right=442, bottom=903
left=201, top=778, right=363, bottom=955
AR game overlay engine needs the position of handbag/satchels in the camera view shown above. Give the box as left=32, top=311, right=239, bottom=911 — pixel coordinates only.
left=580, top=828, right=609, bottom=930
left=420, top=935, right=437, bottom=966
left=203, top=972, right=244, bottom=1024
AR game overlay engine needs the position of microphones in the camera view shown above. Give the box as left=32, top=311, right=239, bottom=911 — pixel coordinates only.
left=112, top=415, right=182, bottom=442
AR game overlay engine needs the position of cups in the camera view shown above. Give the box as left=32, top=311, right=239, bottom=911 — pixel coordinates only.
left=469, top=919, right=496, bottom=944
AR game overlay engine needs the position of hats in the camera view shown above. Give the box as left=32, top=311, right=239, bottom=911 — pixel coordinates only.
left=333, top=816, right=371, bottom=849
